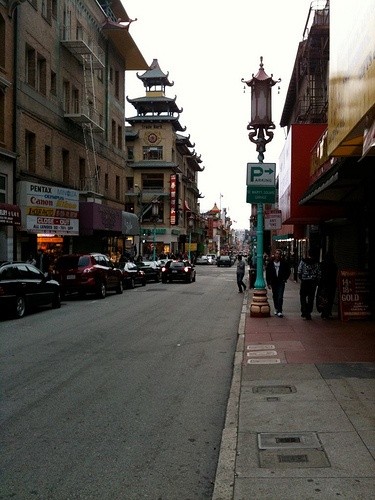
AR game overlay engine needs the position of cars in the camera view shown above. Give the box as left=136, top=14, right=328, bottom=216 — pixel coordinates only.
left=139, top=259, right=196, bottom=284
left=115, top=262, right=146, bottom=289
left=196, top=253, right=238, bottom=268
left=0, top=261, right=62, bottom=318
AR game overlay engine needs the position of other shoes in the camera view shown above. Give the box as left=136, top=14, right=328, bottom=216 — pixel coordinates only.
left=301, top=313, right=305, bottom=317
left=274, top=309, right=277, bottom=315
left=244, top=286, right=246, bottom=291
left=277, top=312, right=283, bottom=317
left=238, top=291, right=243, bottom=293
left=305, top=312, right=312, bottom=320
left=321, top=311, right=333, bottom=319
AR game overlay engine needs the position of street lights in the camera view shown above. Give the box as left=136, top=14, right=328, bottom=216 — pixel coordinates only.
left=189, top=214, right=194, bottom=261
left=151, top=197, right=160, bottom=262
left=241, top=54, right=281, bottom=318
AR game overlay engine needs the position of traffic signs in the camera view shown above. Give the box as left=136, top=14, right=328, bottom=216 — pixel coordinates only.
left=246, top=162, right=277, bottom=185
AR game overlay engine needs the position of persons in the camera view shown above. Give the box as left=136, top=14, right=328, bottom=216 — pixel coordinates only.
left=248, top=252, right=268, bottom=269
left=35, top=248, right=50, bottom=273
left=297, top=248, right=338, bottom=319
left=237, top=255, right=246, bottom=293
left=160, top=251, right=196, bottom=267
left=131, top=244, right=137, bottom=256
left=26, top=255, right=37, bottom=265
left=110, top=250, right=143, bottom=268
left=266, top=249, right=291, bottom=317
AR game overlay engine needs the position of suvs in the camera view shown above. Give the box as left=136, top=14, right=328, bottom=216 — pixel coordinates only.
left=48, top=252, right=123, bottom=298
